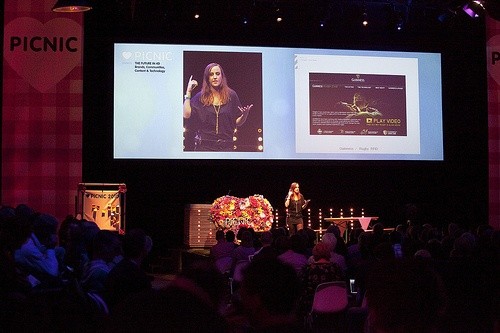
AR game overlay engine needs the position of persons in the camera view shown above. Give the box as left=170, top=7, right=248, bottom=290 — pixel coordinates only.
left=184, top=63, right=254, bottom=151
left=0, top=182, right=500, bottom=333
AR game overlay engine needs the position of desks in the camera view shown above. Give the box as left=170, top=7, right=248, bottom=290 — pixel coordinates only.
left=323, top=217, right=379, bottom=243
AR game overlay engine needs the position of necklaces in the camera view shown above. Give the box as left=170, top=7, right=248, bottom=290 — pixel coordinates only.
left=211, top=103, right=223, bottom=134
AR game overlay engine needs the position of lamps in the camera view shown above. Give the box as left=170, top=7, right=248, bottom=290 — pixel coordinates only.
left=51, top=0, right=93, bottom=13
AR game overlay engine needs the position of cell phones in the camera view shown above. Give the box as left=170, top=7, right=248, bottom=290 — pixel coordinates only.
left=350, top=279, right=357, bottom=294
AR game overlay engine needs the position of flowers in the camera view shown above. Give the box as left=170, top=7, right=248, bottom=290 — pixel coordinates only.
left=118, top=184, right=127, bottom=193
left=206, top=195, right=274, bottom=236
left=79, top=184, right=86, bottom=193
left=119, top=229, right=126, bottom=237
